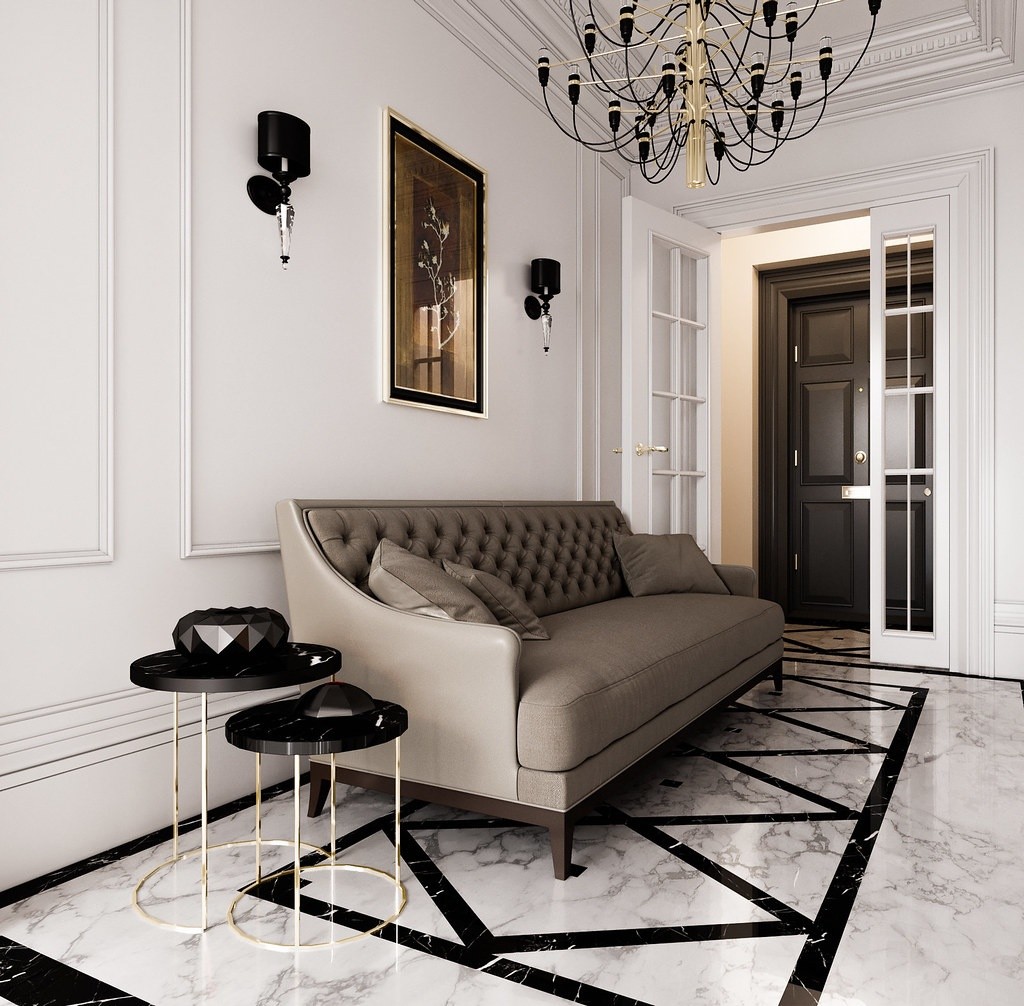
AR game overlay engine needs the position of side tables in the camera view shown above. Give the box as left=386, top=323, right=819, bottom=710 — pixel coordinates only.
left=224, top=699, right=408, bottom=953
left=129, top=641, right=341, bottom=935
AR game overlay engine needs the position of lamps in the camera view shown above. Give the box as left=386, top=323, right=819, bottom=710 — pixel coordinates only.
left=247, top=110, right=311, bottom=271
left=537, top=0, right=882, bottom=190
left=524, top=257, right=561, bottom=356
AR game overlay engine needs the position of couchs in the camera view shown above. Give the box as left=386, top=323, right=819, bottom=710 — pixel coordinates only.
left=274, top=498, right=783, bottom=881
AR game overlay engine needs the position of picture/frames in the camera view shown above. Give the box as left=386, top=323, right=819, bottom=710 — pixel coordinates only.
left=381, top=105, right=489, bottom=419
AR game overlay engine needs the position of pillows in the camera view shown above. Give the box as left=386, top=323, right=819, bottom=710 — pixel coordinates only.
left=611, top=528, right=730, bottom=599
left=368, top=537, right=499, bottom=626
left=441, top=558, right=552, bottom=641
